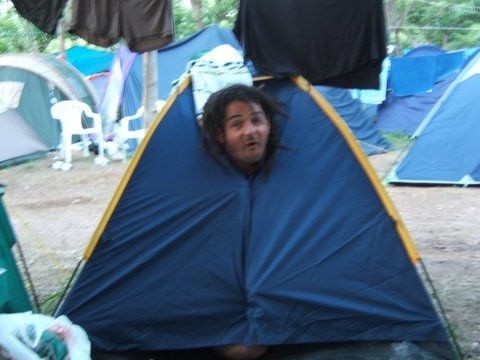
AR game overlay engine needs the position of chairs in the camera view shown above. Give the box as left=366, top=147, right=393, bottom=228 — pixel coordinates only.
left=116, top=100, right=167, bottom=155
left=49, top=100, right=104, bottom=162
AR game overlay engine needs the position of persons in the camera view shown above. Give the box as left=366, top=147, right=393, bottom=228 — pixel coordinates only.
left=201, top=83, right=281, bottom=167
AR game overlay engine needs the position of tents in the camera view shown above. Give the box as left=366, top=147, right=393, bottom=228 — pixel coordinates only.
left=47, top=54, right=469, bottom=360
left=1, top=23, right=479, bottom=188
left=0, top=183, right=44, bottom=318
